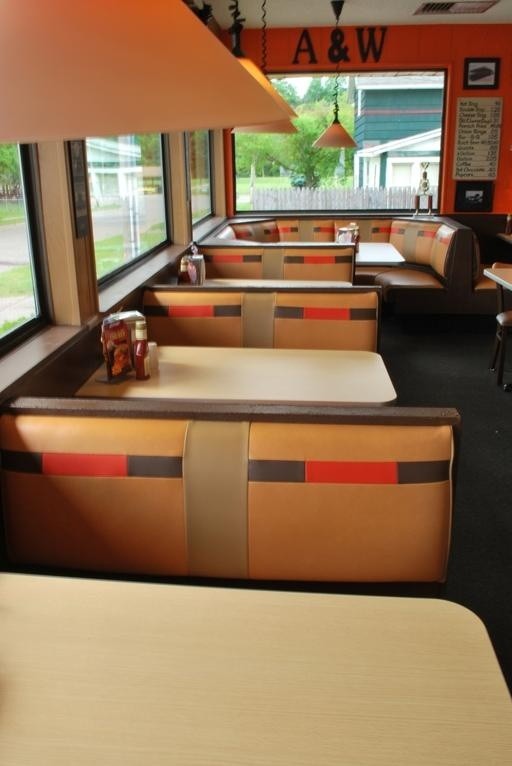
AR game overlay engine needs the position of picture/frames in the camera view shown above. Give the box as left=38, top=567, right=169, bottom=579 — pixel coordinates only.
left=454, top=180, right=495, bottom=212
left=463, top=56, right=501, bottom=90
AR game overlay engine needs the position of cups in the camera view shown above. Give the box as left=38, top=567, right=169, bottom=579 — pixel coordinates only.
left=148, top=343, right=157, bottom=372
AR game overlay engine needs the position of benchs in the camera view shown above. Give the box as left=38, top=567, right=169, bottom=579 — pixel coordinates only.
left=140, top=284, right=381, bottom=352
left=0, top=395, right=462, bottom=583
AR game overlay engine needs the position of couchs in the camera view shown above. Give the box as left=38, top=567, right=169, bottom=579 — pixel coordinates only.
left=199, top=217, right=461, bottom=334
left=446, top=214, right=512, bottom=326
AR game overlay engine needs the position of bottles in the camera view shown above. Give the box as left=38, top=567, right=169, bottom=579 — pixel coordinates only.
left=353, top=227, right=360, bottom=253
left=178, top=261, right=191, bottom=287
left=135, top=320, right=152, bottom=382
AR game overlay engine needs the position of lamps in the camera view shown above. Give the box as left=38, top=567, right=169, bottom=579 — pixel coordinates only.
left=311, top=0, right=359, bottom=147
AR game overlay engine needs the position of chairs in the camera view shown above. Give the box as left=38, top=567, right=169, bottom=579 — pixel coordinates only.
left=490, top=262, right=512, bottom=387
left=197, top=245, right=356, bottom=280
